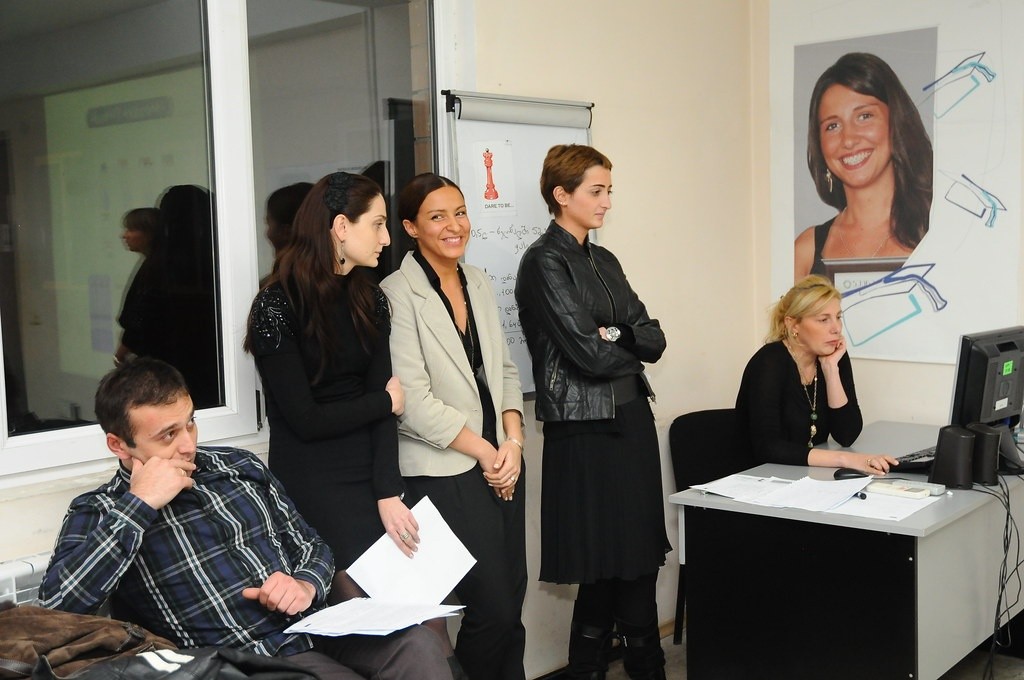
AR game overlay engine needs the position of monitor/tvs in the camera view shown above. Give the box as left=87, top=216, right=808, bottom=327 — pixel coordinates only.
left=950, top=324, right=1024, bottom=475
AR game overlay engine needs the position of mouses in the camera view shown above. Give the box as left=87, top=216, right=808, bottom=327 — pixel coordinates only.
left=833, top=467, right=869, bottom=480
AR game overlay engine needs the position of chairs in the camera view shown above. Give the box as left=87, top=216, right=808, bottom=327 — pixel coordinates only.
left=668, top=408, right=736, bottom=646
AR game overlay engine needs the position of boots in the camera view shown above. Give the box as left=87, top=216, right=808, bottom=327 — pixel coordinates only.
left=566, top=613, right=666, bottom=680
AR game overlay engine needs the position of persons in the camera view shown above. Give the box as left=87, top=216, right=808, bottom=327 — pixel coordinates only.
left=260, top=159, right=391, bottom=277
left=246, top=170, right=463, bottom=679
left=794, top=52, right=933, bottom=288
left=381, top=172, right=531, bottom=680
left=37, top=355, right=336, bottom=679
left=117, top=184, right=225, bottom=409
left=735, top=274, right=899, bottom=476
left=515, top=145, right=674, bottom=680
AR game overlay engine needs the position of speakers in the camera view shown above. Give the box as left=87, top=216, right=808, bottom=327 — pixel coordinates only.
left=928, top=425, right=1001, bottom=489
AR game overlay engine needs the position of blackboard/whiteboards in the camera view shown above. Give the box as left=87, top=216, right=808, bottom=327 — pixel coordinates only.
left=443, top=87, right=597, bottom=397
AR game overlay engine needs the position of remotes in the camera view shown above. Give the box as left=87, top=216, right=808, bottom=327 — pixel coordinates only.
left=893, top=479, right=946, bottom=496
left=866, top=483, right=930, bottom=499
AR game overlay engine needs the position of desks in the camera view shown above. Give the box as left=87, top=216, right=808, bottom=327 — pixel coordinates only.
left=667, top=418, right=1024, bottom=680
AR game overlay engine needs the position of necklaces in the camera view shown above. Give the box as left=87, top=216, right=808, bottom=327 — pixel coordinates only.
left=838, top=206, right=894, bottom=257
left=425, top=259, right=476, bottom=376
left=786, top=337, right=818, bottom=448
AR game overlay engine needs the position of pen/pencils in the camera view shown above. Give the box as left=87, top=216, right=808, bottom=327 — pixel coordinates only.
left=854, top=492, right=867, bottom=500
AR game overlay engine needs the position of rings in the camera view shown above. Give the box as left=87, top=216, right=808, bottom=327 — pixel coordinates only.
left=510, top=476, right=517, bottom=481
left=867, top=458, right=871, bottom=464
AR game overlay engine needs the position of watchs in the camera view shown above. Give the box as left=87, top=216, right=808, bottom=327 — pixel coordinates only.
left=604, top=327, right=621, bottom=342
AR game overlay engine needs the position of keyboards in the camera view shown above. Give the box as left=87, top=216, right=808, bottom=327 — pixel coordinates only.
left=888, top=445, right=937, bottom=471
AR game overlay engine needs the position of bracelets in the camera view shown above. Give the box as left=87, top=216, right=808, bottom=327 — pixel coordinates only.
left=112, top=352, right=124, bottom=366
left=507, top=437, right=524, bottom=449
